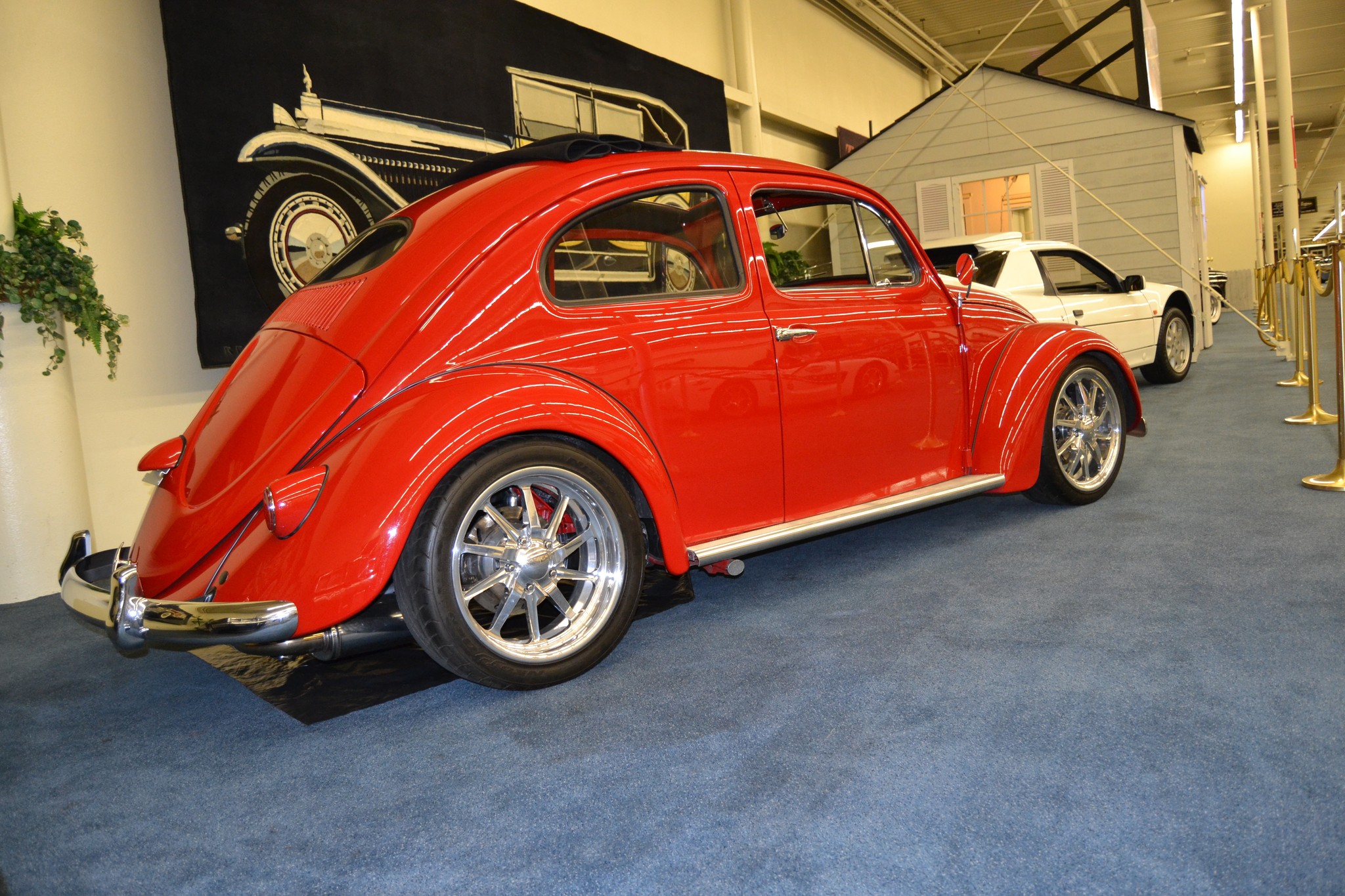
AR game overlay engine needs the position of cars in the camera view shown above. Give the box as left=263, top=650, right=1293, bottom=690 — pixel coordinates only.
left=58, top=130, right=1149, bottom=686
left=1197, top=257, right=1231, bottom=327
left=871, top=228, right=1198, bottom=385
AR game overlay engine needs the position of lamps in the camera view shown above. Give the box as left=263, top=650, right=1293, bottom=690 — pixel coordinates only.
left=1234, top=106, right=1244, bottom=144
left=1230, top=0, right=1243, bottom=107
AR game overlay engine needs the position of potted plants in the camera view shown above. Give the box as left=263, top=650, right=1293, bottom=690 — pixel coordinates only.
left=0, top=191, right=130, bottom=379
